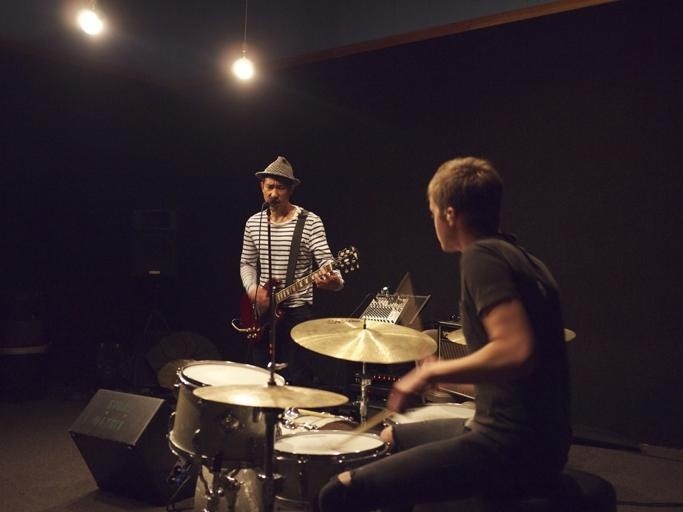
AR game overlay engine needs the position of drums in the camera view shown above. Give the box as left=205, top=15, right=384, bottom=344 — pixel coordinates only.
left=168, top=361, right=286, bottom=464
left=273, top=409, right=359, bottom=436
left=389, top=403, right=477, bottom=447
left=271, top=430, right=392, bottom=508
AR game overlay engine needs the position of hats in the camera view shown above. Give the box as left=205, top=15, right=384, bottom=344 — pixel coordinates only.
left=256, top=156, right=300, bottom=184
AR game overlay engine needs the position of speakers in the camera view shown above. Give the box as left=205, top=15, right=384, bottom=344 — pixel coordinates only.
left=68, top=388, right=174, bottom=504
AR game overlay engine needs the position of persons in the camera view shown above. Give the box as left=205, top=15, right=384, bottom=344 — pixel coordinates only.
left=239, top=156, right=345, bottom=387
left=318, top=157, right=572, bottom=512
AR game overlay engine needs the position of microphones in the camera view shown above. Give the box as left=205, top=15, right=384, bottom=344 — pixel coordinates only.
left=262, top=197, right=278, bottom=211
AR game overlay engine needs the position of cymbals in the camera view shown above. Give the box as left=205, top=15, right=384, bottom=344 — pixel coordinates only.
left=193, top=386, right=349, bottom=410
left=290, top=317, right=439, bottom=363
left=447, top=326, right=576, bottom=346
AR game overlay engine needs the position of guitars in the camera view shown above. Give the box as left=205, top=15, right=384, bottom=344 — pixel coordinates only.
left=237, top=246, right=360, bottom=343
